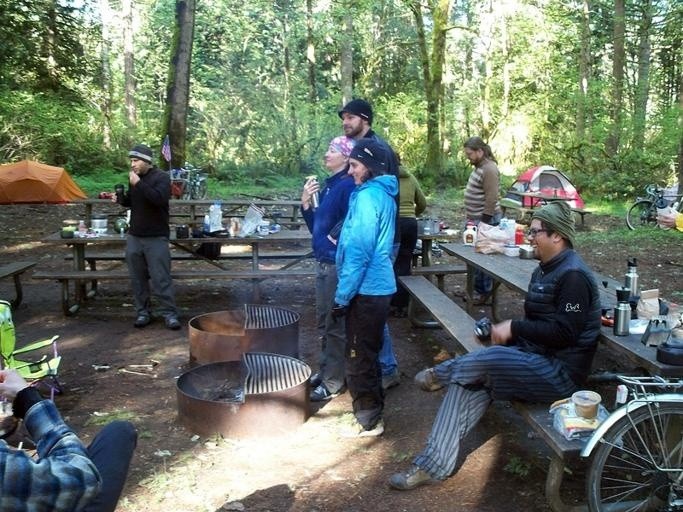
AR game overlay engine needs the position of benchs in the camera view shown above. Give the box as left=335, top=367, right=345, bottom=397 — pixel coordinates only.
left=0, top=261, right=37, bottom=306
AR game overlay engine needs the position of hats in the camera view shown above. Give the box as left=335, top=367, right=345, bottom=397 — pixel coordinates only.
left=128, top=145, right=153, bottom=164
left=329, top=99, right=388, bottom=174
left=531, top=200, right=576, bottom=249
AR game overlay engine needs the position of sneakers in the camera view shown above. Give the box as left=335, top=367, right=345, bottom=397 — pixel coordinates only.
left=454, top=279, right=501, bottom=306
left=414, top=367, right=446, bottom=393
left=309, top=369, right=401, bottom=437
left=389, top=465, right=434, bottom=490
left=165, top=318, right=181, bottom=329
left=133, top=315, right=151, bottom=327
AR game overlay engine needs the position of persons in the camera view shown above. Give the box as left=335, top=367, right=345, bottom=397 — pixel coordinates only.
left=297, top=134, right=356, bottom=402
left=388, top=201, right=602, bottom=490
left=110, top=146, right=180, bottom=329
left=390, top=150, right=428, bottom=319
left=336, top=98, right=400, bottom=396
left=654, top=207, right=682, bottom=232
left=0, top=367, right=136, bottom=512
left=453, top=137, right=500, bottom=306
left=332, top=136, right=401, bottom=439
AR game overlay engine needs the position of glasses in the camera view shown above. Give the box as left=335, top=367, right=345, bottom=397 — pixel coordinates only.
left=527, top=229, right=550, bottom=238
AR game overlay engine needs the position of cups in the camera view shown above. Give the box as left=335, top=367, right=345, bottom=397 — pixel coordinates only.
left=175, top=225, right=189, bottom=239
left=473, top=317, right=494, bottom=347
left=90, top=219, right=107, bottom=231
left=257, top=221, right=270, bottom=236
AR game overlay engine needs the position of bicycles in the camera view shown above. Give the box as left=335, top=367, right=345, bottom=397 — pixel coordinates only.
left=169, top=168, right=208, bottom=198
left=578, top=375, right=682, bottom=512
left=624, top=186, right=683, bottom=231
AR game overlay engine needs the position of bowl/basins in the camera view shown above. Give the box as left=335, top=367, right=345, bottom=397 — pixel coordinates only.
left=572, top=390, right=603, bottom=419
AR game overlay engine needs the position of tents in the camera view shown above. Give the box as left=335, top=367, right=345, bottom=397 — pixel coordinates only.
left=0, top=161, right=87, bottom=203
left=505, top=165, right=583, bottom=209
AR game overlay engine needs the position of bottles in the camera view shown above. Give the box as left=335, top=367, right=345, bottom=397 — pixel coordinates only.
left=614, top=288, right=630, bottom=336
left=462, top=221, right=477, bottom=245
left=209, top=200, right=222, bottom=233
left=416, top=218, right=439, bottom=236
left=625, top=258, right=639, bottom=295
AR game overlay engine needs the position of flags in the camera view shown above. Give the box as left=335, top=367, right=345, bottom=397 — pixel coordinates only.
left=161, top=135, right=171, bottom=162
